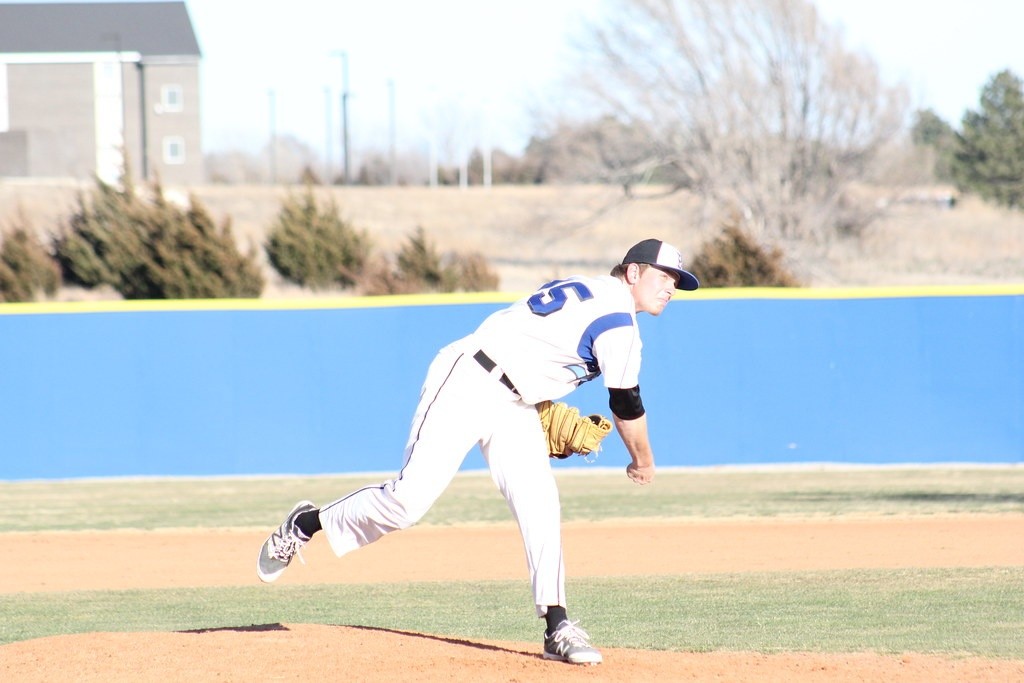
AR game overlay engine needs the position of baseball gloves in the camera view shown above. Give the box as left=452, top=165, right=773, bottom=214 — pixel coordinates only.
left=535, top=400, right=613, bottom=463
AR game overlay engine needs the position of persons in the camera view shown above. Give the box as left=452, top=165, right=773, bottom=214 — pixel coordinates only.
left=258, top=236, right=700, bottom=664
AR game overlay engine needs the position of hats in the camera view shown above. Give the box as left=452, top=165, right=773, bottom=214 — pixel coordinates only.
left=622, top=238, right=698, bottom=291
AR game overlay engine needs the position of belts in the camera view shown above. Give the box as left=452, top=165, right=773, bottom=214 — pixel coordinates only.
left=473, top=350, right=518, bottom=395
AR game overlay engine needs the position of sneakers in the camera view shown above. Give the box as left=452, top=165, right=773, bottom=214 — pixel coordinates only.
left=544, top=617, right=603, bottom=663
left=256, top=499, right=319, bottom=583
